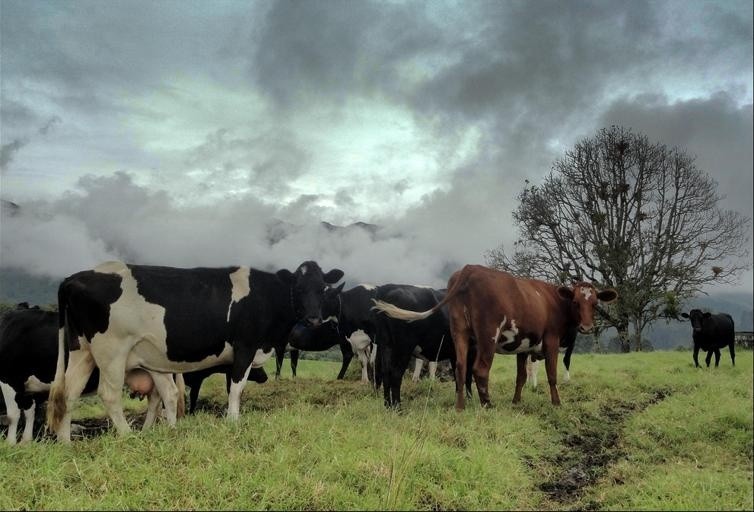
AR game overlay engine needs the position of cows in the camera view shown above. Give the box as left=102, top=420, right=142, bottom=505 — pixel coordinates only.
left=681, top=309, right=736, bottom=368
left=0, top=261, right=619, bottom=448
